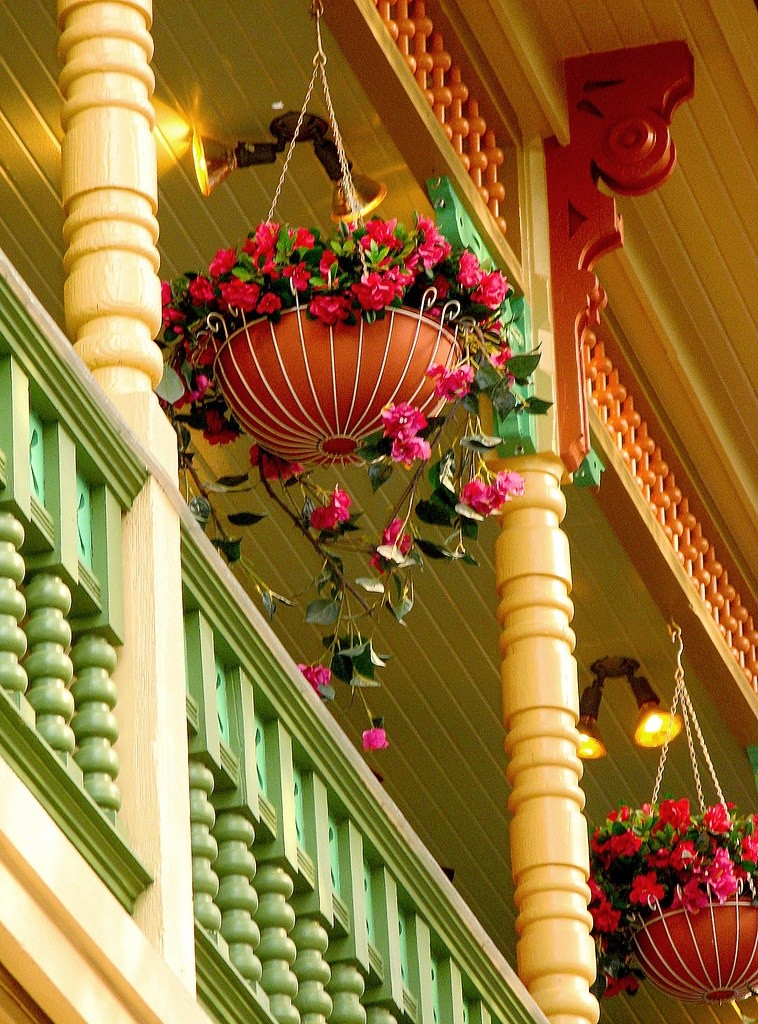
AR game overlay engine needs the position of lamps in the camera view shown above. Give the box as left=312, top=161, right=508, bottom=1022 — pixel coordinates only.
left=568, top=654, right=681, bottom=759
left=193, top=112, right=388, bottom=225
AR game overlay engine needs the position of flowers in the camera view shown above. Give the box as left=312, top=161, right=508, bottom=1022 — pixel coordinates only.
left=158, top=213, right=555, bottom=751
left=588, top=790, right=758, bottom=998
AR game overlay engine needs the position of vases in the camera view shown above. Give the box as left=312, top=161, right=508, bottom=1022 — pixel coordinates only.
left=619, top=884, right=758, bottom=1003
left=191, top=263, right=477, bottom=467
